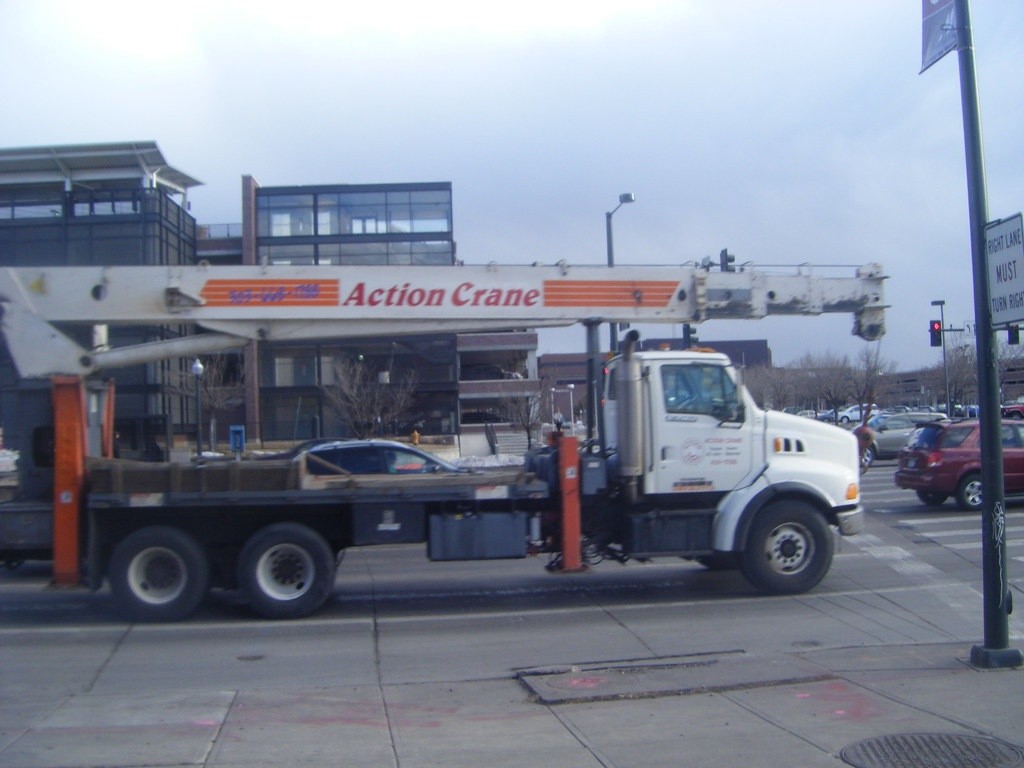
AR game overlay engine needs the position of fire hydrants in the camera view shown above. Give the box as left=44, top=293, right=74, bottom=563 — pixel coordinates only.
left=410, top=430, right=420, bottom=447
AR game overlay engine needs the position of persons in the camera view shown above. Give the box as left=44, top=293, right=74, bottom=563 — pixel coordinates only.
left=554, top=408, right=563, bottom=431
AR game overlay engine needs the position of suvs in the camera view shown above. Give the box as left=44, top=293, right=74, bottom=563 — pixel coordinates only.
left=896, top=416, right=1024, bottom=511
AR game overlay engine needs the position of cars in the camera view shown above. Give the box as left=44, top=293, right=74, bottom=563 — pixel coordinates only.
left=783, top=402, right=1024, bottom=469
left=249, top=436, right=478, bottom=483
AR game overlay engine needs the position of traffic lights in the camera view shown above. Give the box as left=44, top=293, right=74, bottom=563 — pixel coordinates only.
left=930, top=319, right=942, bottom=346
left=720, top=248, right=736, bottom=273
left=683, top=322, right=699, bottom=349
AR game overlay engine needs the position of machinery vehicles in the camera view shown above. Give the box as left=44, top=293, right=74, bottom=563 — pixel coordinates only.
left=0, top=263, right=894, bottom=625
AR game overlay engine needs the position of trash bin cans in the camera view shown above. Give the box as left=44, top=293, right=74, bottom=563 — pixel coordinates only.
left=170, top=448, right=192, bottom=464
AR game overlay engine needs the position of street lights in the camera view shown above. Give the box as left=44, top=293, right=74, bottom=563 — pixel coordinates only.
left=549, top=386, right=555, bottom=425
left=930, top=300, right=951, bottom=418
left=567, top=383, right=575, bottom=435
left=606, top=192, right=637, bottom=352
